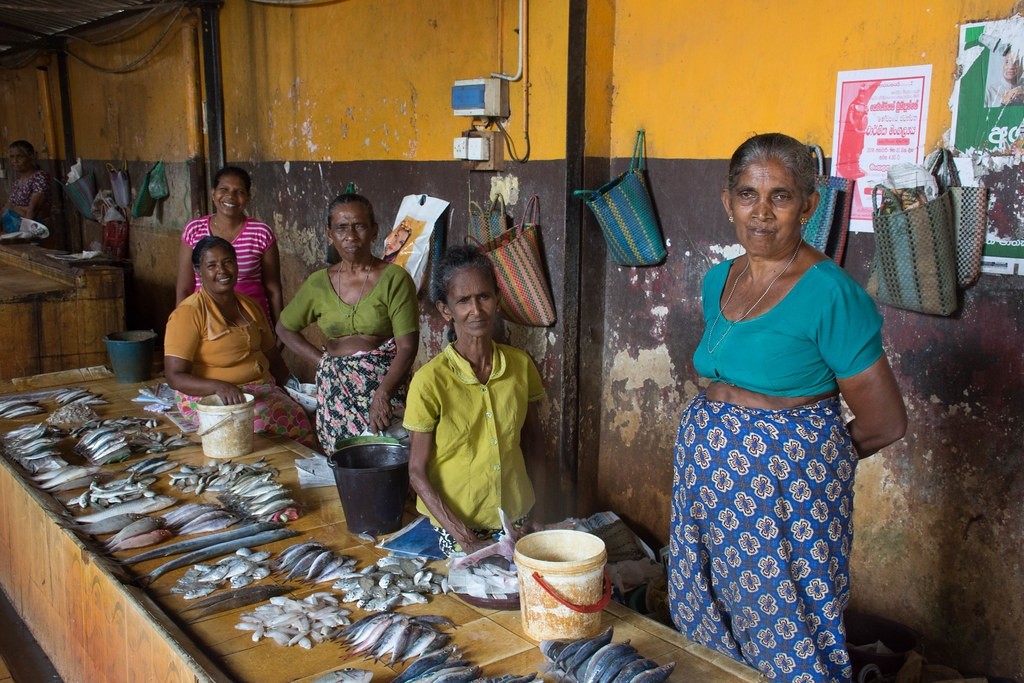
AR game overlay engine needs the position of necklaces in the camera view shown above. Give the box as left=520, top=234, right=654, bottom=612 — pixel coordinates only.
left=337, top=258, right=374, bottom=316
left=706, top=237, right=803, bottom=355
left=214, top=217, right=242, bottom=241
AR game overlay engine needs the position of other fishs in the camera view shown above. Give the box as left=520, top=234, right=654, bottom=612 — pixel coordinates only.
left=0, top=385, right=677, bottom=683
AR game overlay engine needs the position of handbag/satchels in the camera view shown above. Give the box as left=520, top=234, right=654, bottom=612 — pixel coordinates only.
left=100, top=206, right=128, bottom=261
left=464, top=195, right=558, bottom=327
left=149, top=156, right=169, bottom=199
left=924, top=146, right=990, bottom=286
left=324, top=182, right=355, bottom=266
left=574, top=130, right=668, bottom=266
left=466, top=193, right=507, bottom=246
left=382, top=193, right=450, bottom=303
left=800, top=144, right=854, bottom=266
left=132, top=161, right=157, bottom=219
left=105, top=159, right=130, bottom=210
left=865, top=184, right=958, bottom=317
left=53, top=161, right=96, bottom=220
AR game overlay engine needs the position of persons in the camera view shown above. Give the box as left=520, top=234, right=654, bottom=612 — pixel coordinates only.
left=175, top=166, right=284, bottom=327
left=402, top=253, right=548, bottom=554
left=276, top=193, right=420, bottom=453
left=164, top=236, right=313, bottom=446
left=667, top=130, right=909, bottom=683
left=1, top=140, right=56, bottom=249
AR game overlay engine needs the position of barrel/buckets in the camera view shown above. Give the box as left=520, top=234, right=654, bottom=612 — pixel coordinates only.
left=334, top=437, right=400, bottom=450
left=195, top=393, right=256, bottom=459
left=102, top=330, right=157, bottom=383
left=327, top=442, right=411, bottom=536
left=511, top=529, right=611, bottom=642
